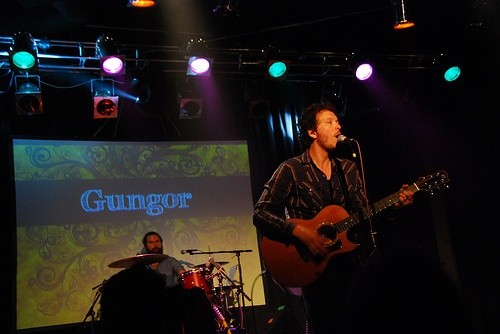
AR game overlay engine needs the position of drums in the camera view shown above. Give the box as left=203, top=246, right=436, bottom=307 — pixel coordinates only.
left=181, top=269, right=215, bottom=301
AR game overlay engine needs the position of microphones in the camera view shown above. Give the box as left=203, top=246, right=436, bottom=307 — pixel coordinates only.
left=338, top=135, right=354, bottom=144
left=181, top=249, right=198, bottom=254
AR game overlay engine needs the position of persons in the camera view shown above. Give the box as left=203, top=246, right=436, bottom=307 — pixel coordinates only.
left=139, top=231, right=185, bottom=287
left=252, top=103, right=415, bottom=334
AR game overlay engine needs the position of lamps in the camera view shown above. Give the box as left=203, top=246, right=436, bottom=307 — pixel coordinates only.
left=176, top=79, right=203, bottom=119
left=389, top=0, right=417, bottom=30
left=90, top=76, right=119, bottom=119
left=211, top=0, right=241, bottom=17
left=345, top=50, right=374, bottom=81
left=298, top=54, right=328, bottom=80
left=14, top=75, right=43, bottom=116
left=238, top=80, right=271, bottom=118
left=186, top=36, right=214, bottom=77
left=322, top=80, right=347, bottom=115
left=8, top=32, right=39, bottom=73
left=262, top=46, right=287, bottom=81
left=428, top=49, right=461, bottom=82
left=95, top=35, right=128, bottom=76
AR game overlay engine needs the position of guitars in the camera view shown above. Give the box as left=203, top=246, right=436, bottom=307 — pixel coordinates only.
left=259, top=171, right=449, bottom=289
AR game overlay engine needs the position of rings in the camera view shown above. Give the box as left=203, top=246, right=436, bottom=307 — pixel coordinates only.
left=404, top=198, right=408, bottom=201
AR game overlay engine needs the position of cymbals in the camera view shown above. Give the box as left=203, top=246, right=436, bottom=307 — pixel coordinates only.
left=194, top=261, right=229, bottom=269
left=108, top=253, right=168, bottom=269
